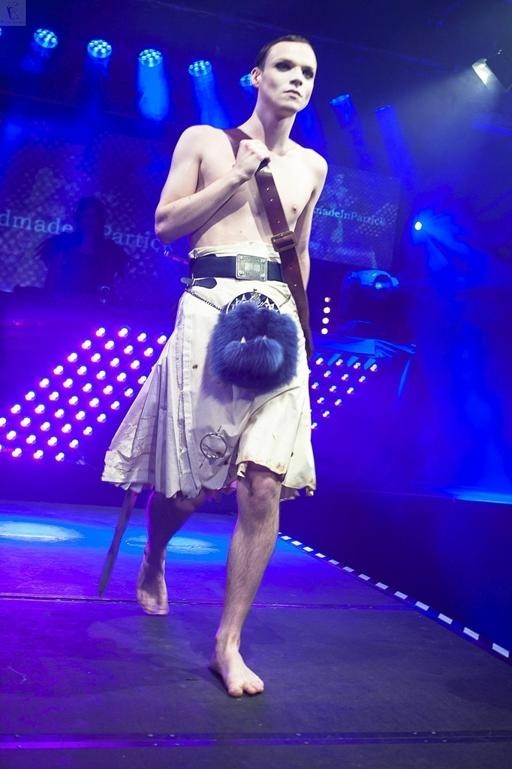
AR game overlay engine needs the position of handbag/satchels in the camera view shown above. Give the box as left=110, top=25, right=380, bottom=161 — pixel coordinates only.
left=212, top=291, right=296, bottom=388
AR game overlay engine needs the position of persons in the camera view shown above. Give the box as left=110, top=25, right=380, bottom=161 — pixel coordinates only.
left=100, top=33, right=332, bottom=700
left=37, top=195, right=126, bottom=305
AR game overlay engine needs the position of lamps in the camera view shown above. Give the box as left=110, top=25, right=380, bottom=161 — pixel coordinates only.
left=468, top=51, right=510, bottom=98
